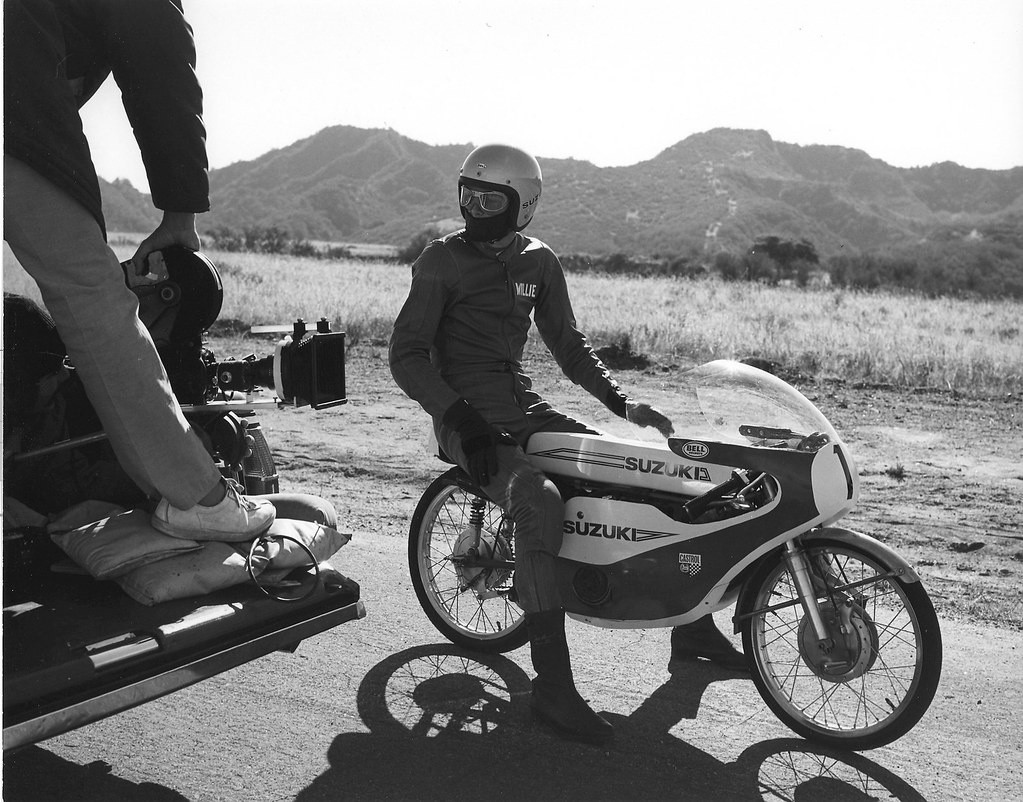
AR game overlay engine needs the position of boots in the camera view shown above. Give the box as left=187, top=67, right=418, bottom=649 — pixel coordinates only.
left=525, top=606, right=617, bottom=746
left=670, top=613, right=749, bottom=672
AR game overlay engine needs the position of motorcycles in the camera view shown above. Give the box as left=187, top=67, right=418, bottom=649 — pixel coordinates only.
left=407, top=355, right=942, bottom=751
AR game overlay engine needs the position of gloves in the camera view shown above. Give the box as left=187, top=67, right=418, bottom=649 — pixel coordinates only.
left=606, top=386, right=676, bottom=440
left=443, top=396, right=519, bottom=487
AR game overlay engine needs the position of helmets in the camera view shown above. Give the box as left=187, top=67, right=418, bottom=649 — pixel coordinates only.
left=458, top=143, right=542, bottom=232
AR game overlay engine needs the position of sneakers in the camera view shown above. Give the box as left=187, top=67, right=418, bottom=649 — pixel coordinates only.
left=151, top=475, right=276, bottom=543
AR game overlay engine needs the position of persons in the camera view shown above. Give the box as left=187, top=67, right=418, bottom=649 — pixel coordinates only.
left=3, top=0, right=275, bottom=542
left=390, top=145, right=747, bottom=741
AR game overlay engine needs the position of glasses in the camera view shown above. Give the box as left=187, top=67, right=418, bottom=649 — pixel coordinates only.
left=460, top=185, right=511, bottom=212
